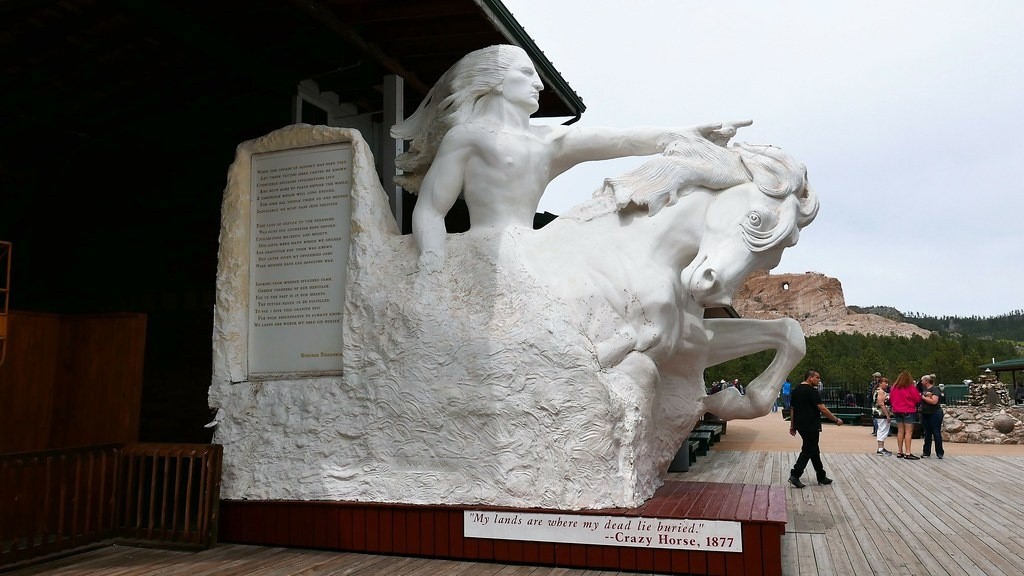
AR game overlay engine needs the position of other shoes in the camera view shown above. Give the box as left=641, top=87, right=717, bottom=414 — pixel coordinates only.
left=873, top=434, right=877, bottom=437
left=937, top=455, right=943, bottom=459
left=921, top=454, right=930, bottom=458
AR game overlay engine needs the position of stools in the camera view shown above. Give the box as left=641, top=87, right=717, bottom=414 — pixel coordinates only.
left=696, top=425, right=722, bottom=443
left=687, top=441, right=700, bottom=466
left=689, top=432, right=711, bottom=456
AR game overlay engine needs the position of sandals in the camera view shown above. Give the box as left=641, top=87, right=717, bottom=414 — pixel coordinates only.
left=897, top=453, right=920, bottom=460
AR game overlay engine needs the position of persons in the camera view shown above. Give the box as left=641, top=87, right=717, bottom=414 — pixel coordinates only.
left=390, top=45, right=753, bottom=253
left=873, top=377, right=892, bottom=456
left=711, top=379, right=744, bottom=395
left=771, top=390, right=780, bottom=413
left=868, top=372, right=882, bottom=437
left=889, top=372, right=921, bottom=460
left=919, top=374, right=944, bottom=459
left=787, top=369, right=843, bottom=489
left=782, top=379, right=791, bottom=410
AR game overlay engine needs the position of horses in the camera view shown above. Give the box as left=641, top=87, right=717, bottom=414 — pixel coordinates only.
left=474, top=127, right=821, bottom=419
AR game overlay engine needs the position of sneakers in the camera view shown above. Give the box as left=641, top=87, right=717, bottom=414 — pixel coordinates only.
left=877, top=448, right=892, bottom=457
left=817, top=477, right=833, bottom=486
left=787, top=476, right=807, bottom=488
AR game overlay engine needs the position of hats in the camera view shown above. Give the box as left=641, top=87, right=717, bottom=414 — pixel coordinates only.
left=872, top=371, right=881, bottom=377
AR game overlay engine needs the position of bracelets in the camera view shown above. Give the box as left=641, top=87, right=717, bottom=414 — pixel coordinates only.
left=836, top=418, right=838, bottom=423
left=791, top=426, right=794, bottom=427
